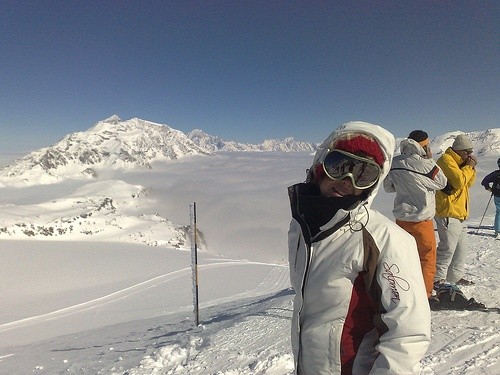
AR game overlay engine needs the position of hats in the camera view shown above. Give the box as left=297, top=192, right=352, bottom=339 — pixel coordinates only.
left=313, top=134, right=384, bottom=193
left=452, top=135, right=473, bottom=150
left=408, top=130, right=429, bottom=148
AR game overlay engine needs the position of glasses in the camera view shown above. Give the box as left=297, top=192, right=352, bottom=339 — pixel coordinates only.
left=465, top=150, right=473, bottom=154
left=318, top=148, right=381, bottom=189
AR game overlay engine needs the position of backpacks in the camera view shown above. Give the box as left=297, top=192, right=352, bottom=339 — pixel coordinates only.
left=429, top=283, right=487, bottom=311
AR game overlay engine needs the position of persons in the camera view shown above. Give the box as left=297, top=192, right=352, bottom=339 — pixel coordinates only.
left=383, top=130, right=448, bottom=299
left=481, top=158, right=500, bottom=238
left=287, top=121, right=431, bottom=375
left=436, top=135, right=475, bottom=290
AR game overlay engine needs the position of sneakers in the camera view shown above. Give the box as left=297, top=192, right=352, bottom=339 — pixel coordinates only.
left=433, top=279, right=451, bottom=289
left=456, top=278, right=471, bottom=286
left=495, top=232, right=500, bottom=239
